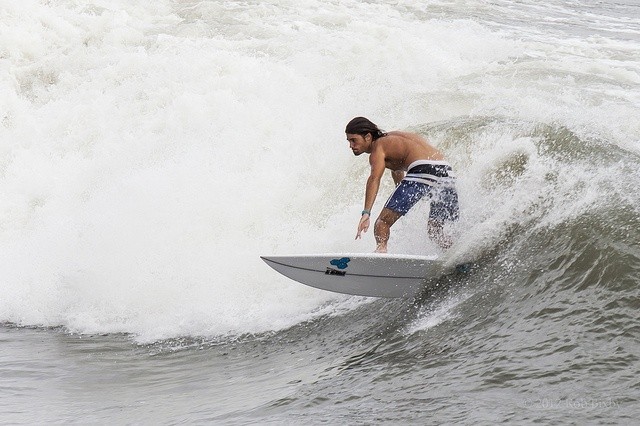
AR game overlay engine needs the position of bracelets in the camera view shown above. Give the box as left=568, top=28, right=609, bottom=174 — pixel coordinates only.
left=362, top=210, right=370, bottom=216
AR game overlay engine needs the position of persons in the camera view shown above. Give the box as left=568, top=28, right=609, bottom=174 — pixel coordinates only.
left=345, top=117, right=460, bottom=253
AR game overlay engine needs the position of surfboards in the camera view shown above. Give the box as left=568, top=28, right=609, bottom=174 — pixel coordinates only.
left=259, top=252, right=437, bottom=299
